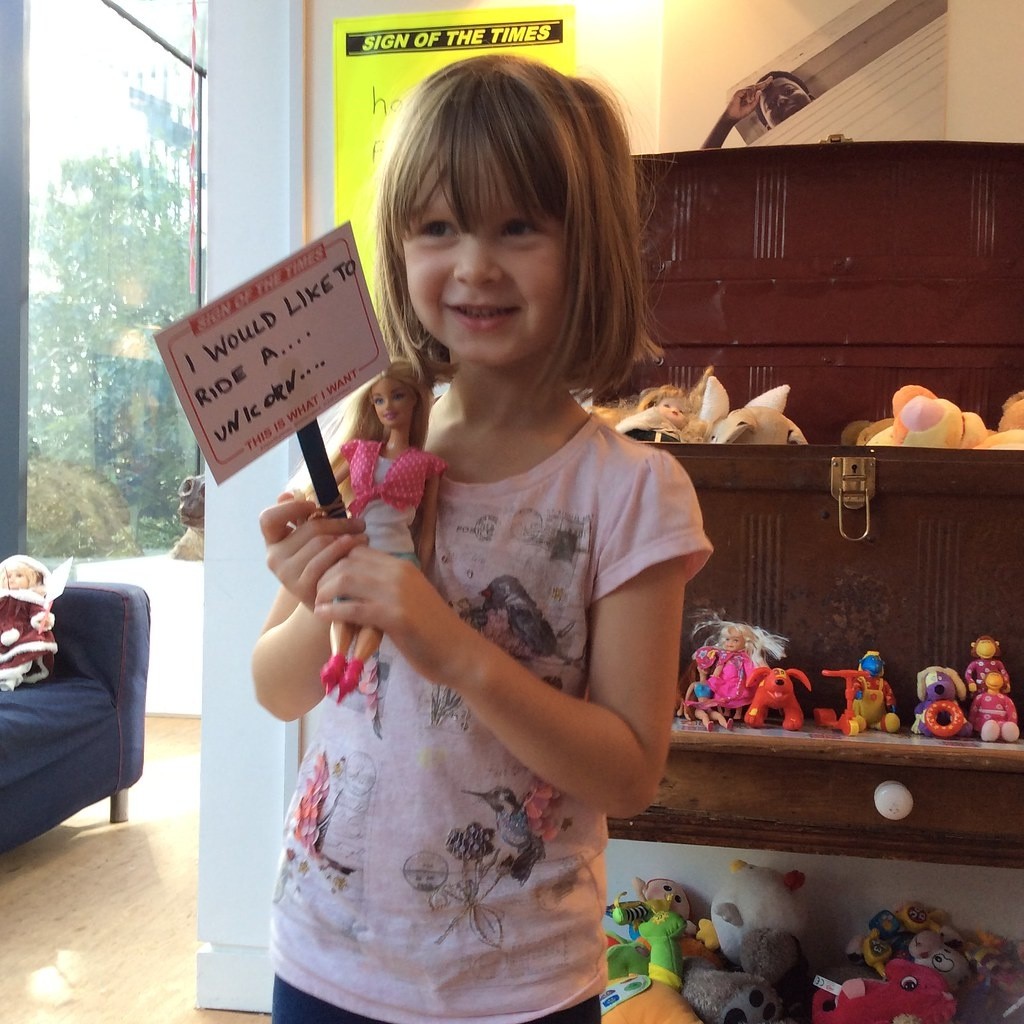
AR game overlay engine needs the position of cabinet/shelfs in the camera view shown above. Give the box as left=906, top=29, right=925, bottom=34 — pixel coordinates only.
left=560, top=139, right=1024, bottom=1022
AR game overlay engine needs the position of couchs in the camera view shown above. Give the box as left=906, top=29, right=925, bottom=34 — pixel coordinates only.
left=0, top=580, right=155, bottom=855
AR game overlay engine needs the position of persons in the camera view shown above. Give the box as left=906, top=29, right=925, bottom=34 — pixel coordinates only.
left=682, top=658, right=733, bottom=730
left=615, top=382, right=694, bottom=447
left=0, top=554, right=59, bottom=691
left=252, top=57, right=713, bottom=1023
left=687, top=606, right=790, bottom=724
left=319, top=356, right=445, bottom=704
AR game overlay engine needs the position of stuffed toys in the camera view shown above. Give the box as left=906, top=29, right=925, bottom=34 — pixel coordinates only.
left=591, top=636, right=1024, bottom=1024
left=699, top=375, right=1024, bottom=451
